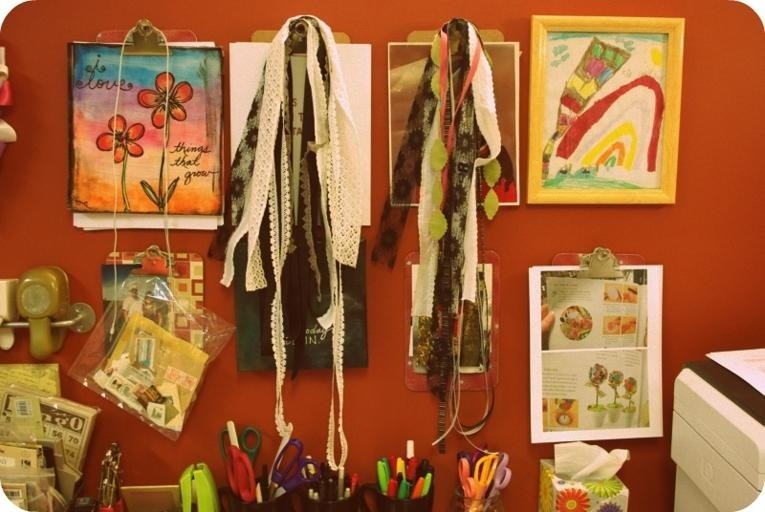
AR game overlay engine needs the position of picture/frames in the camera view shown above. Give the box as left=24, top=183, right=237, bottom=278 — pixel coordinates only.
left=526, top=14, right=686, bottom=204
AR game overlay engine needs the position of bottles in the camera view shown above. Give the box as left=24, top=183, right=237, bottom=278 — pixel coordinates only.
left=450, top=487, right=504, bottom=512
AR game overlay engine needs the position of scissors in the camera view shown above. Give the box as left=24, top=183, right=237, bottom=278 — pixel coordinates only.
left=220, top=420, right=321, bottom=505
left=458, top=443, right=512, bottom=512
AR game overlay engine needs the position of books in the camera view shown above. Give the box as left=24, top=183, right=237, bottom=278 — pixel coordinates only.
left=0, top=381, right=104, bottom=512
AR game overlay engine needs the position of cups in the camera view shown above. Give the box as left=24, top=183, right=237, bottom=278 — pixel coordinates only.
left=216, top=479, right=435, bottom=512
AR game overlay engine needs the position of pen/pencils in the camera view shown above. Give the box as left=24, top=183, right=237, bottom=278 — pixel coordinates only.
left=376, top=439, right=435, bottom=499
left=301, top=456, right=359, bottom=503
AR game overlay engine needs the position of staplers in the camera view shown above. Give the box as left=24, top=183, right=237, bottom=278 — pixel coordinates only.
left=179, top=463, right=222, bottom=511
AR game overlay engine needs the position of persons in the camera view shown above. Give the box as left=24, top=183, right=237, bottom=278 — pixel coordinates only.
left=542, top=301, right=556, bottom=338
left=121, top=282, right=145, bottom=319
left=100, top=440, right=124, bottom=508
left=143, top=289, right=165, bottom=329
